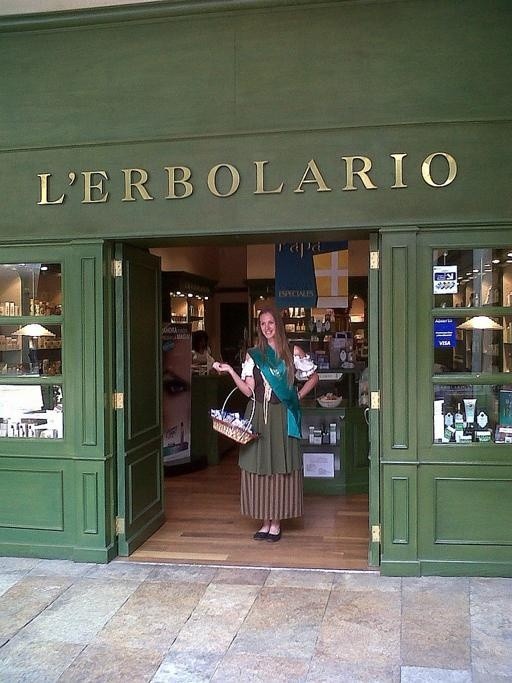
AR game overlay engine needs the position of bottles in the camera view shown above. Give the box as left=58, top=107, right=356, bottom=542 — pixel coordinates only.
left=444, top=401, right=463, bottom=429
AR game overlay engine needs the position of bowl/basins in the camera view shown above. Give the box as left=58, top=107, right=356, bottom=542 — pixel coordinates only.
left=317, top=396, right=342, bottom=407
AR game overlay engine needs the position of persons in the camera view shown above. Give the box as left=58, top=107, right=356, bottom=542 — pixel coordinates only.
left=212, top=305, right=320, bottom=542
left=192, top=330, right=212, bottom=370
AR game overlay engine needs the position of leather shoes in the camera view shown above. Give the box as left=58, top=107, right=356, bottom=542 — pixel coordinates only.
left=253, top=530, right=267, bottom=540
left=266, top=528, right=280, bottom=541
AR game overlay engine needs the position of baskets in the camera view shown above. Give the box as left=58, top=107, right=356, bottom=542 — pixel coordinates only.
left=210, top=385, right=257, bottom=445
